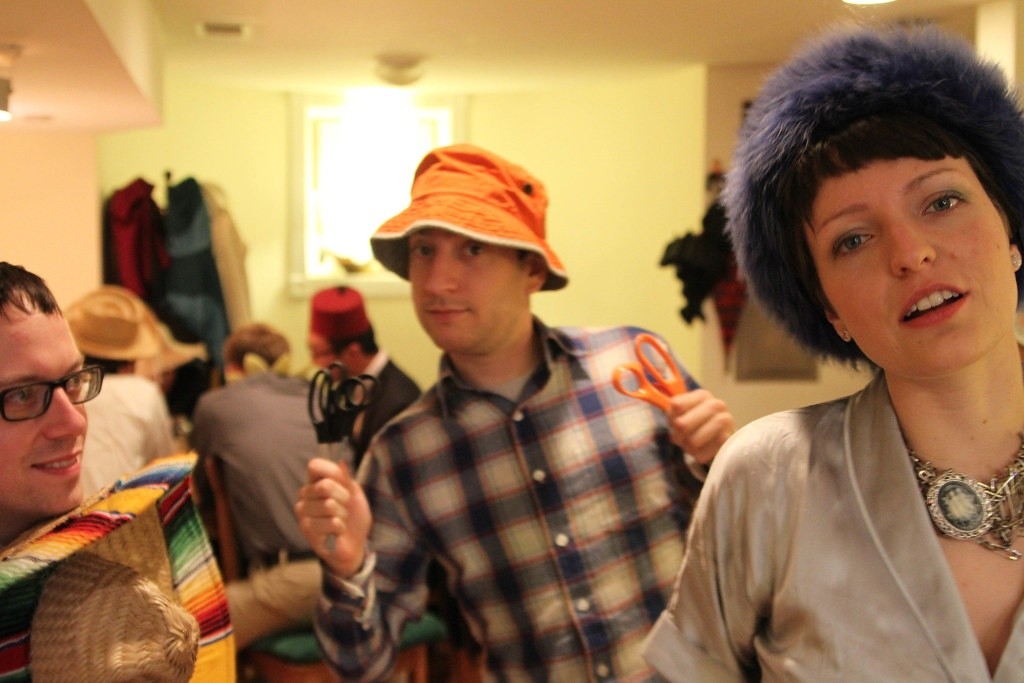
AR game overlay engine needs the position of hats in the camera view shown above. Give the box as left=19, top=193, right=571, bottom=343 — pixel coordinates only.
left=309, top=287, right=370, bottom=341
left=63, top=287, right=161, bottom=360
left=0, top=451, right=237, bottom=683
left=370, top=143, right=569, bottom=293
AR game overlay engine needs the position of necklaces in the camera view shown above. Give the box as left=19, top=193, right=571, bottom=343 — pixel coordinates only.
left=906, top=432, right=1024, bottom=562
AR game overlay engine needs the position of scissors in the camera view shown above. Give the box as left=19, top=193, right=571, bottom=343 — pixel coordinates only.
left=611, top=333, right=690, bottom=418
left=304, top=362, right=383, bottom=466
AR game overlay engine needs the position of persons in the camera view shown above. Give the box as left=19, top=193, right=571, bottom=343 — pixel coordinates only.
left=0, top=260, right=89, bottom=546
left=308, top=287, right=422, bottom=472
left=296, top=144, right=736, bottom=683
left=186, top=323, right=355, bottom=566
left=61, top=283, right=205, bottom=497
left=621, top=22, right=1024, bottom=683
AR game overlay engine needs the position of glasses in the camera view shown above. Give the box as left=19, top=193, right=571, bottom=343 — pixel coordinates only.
left=0, top=365, right=104, bottom=423
